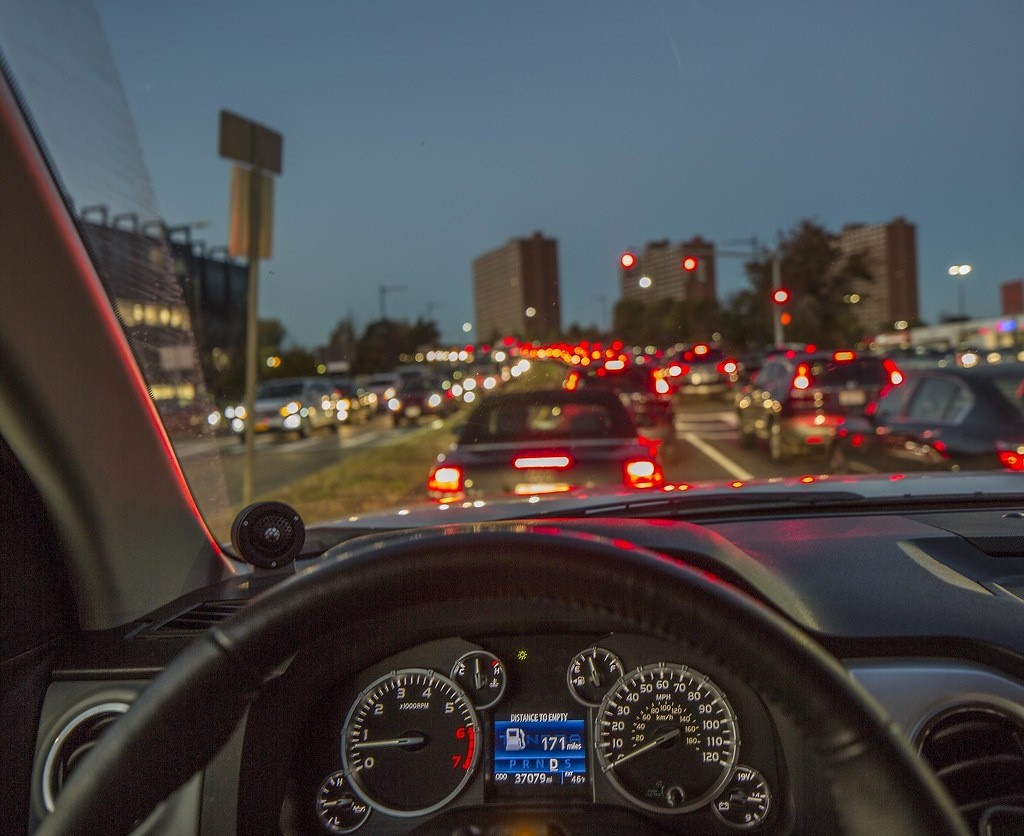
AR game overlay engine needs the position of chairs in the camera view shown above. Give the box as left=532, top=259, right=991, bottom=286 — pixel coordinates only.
left=497, top=411, right=529, bottom=432
left=571, top=412, right=610, bottom=433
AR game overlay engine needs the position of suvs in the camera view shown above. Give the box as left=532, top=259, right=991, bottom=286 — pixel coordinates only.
left=738, top=352, right=904, bottom=462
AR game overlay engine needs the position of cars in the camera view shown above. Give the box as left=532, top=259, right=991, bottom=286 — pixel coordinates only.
left=231, top=376, right=345, bottom=444
left=431, top=337, right=743, bottom=437
left=424, top=385, right=666, bottom=510
left=832, top=363, right=1024, bottom=474
left=345, top=353, right=513, bottom=421
